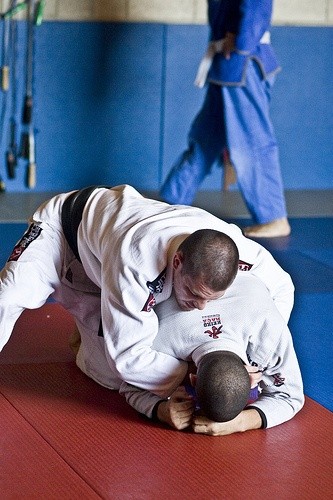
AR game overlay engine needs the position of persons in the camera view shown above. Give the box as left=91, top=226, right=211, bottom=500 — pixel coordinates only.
left=0, top=184, right=305, bottom=437
left=160, top=0, right=291, bottom=238
